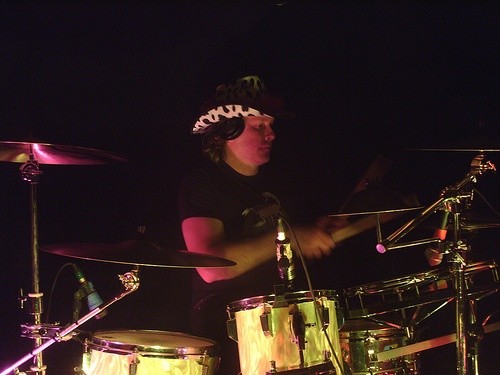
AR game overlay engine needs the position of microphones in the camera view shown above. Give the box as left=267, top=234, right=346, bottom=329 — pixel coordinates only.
left=426, top=210, right=450, bottom=266
left=275, top=220, right=296, bottom=291
left=73, top=265, right=109, bottom=320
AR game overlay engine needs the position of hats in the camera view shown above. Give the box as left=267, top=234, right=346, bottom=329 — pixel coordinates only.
left=192, top=75, right=286, bottom=135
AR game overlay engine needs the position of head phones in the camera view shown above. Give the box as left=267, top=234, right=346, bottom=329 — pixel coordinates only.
left=220, top=116, right=246, bottom=140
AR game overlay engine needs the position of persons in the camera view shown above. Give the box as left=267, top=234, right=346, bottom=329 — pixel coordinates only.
left=181, top=75, right=354, bottom=375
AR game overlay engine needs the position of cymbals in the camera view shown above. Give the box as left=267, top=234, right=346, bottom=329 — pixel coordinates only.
left=0, top=140, right=133, bottom=165
left=406, top=147, right=500, bottom=154
left=325, top=206, right=426, bottom=217
left=420, top=220, right=500, bottom=232
left=38, top=239, right=237, bottom=268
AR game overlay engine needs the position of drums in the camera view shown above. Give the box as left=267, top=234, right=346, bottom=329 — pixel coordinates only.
left=225, top=289, right=344, bottom=375
left=338, top=322, right=422, bottom=375
left=341, top=258, right=500, bottom=320
left=77, top=328, right=222, bottom=375
